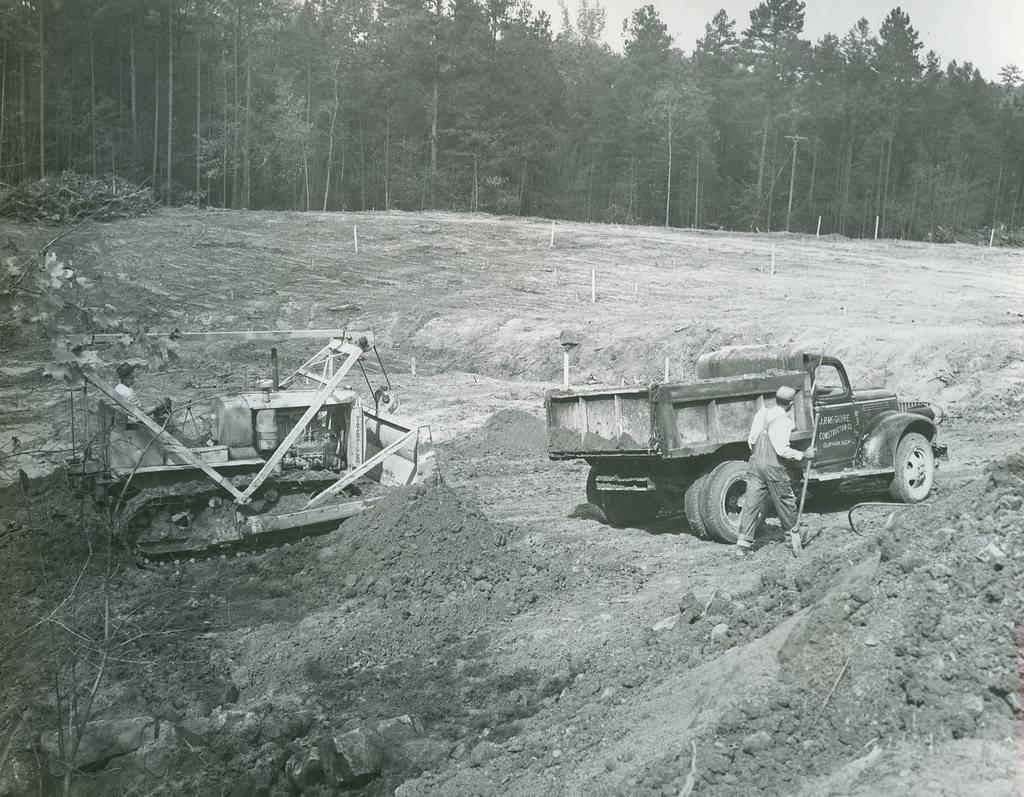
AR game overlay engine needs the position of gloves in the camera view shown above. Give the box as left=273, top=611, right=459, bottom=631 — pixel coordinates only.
left=156, top=399, right=171, bottom=412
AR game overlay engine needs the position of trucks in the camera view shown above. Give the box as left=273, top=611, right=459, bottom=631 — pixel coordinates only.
left=545, top=340, right=952, bottom=543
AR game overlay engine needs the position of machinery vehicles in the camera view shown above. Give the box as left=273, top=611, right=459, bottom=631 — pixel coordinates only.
left=64, top=327, right=441, bottom=568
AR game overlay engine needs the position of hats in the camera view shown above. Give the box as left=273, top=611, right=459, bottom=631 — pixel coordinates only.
left=775, top=386, right=796, bottom=400
left=116, top=362, right=136, bottom=378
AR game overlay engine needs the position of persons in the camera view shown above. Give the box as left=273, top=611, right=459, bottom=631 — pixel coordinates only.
left=113, top=362, right=165, bottom=424
left=736, top=386, right=817, bottom=558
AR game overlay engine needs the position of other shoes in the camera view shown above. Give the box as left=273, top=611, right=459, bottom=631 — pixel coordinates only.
left=735, top=549, right=754, bottom=559
